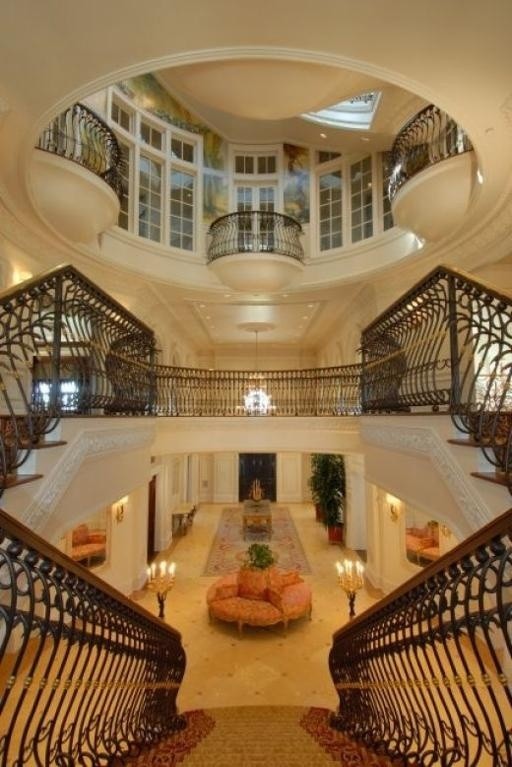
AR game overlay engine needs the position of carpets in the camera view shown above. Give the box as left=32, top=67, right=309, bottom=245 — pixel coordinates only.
left=205, top=505, right=314, bottom=574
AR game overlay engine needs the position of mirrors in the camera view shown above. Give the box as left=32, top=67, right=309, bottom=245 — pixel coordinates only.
left=66, top=505, right=112, bottom=575
left=397, top=500, right=445, bottom=573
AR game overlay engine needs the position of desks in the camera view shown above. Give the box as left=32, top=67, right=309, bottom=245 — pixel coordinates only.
left=241, top=499, right=273, bottom=541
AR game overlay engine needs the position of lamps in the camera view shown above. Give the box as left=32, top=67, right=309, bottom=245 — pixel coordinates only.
left=144, top=560, right=177, bottom=618
left=335, top=558, right=368, bottom=619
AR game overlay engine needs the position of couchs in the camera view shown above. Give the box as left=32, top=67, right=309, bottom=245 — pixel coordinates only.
left=72, top=524, right=106, bottom=567
left=206, top=568, right=313, bottom=639
left=405, top=520, right=440, bottom=565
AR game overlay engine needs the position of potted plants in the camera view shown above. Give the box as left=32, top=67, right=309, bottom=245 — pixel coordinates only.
left=307, top=452, right=346, bottom=545
